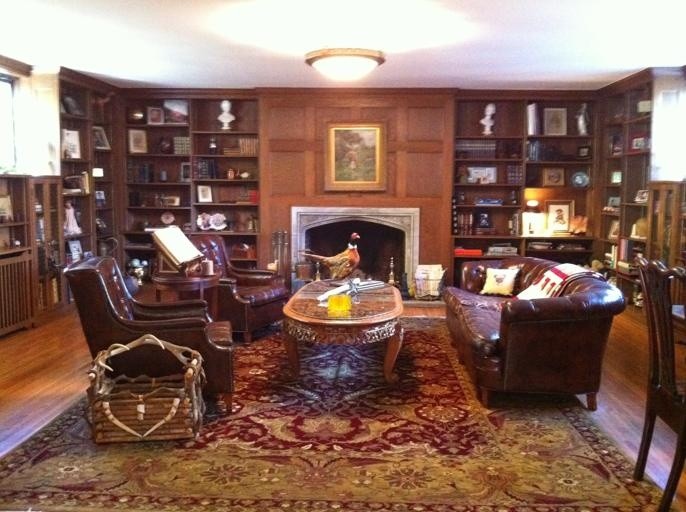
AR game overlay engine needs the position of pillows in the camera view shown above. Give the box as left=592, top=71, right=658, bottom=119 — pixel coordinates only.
left=479, top=268, right=520, bottom=296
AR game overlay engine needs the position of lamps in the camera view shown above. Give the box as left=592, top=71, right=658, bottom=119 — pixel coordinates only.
left=325, top=122, right=388, bottom=191
left=305, top=48, right=385, bottom=80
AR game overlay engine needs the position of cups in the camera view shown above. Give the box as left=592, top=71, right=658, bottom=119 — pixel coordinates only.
left=200, top=260, right=213, bottom=275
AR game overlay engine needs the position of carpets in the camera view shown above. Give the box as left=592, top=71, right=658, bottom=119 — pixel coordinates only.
left=0, top=316, right=686, bottom=512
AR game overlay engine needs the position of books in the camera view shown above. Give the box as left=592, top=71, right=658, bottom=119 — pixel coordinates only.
left=526, top=103, right=540, bottom=138
left=543, top=107, right=570, bottom=136
left=152, top=222, right=205, bottom=267
left=451, top=139, right=587, bottom=256
left=328, top=278, right=384, bottom=293
left=127, top=136, right=259, bottom=207
left=603, top=239, right=640, bottom=275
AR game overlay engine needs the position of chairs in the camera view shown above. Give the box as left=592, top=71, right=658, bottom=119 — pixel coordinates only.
left=641, top=257, right=686, bottom=512
left=177, top=235, right=289, bottom=344
left=63, top=256, right=233, bottom=412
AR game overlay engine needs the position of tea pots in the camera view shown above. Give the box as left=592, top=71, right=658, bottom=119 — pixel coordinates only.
left=224, top=168, right=238, bottom=180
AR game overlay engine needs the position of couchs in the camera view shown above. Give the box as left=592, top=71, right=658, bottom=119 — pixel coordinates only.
left=442, top=256, right=626, bottom=409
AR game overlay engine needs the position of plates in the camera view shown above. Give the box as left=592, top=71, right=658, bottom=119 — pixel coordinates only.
left=160, top=211, right=174, bottom=224
left=208, top=213, right=227, bottom=230
left=196, top=213, right=210, bottom=231
left=570, top=172, right=588, bottom=188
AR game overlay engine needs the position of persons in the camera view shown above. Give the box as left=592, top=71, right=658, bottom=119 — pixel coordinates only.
left=152, top=109, right=162, bottom=121
left=216, top=99, right=237, bottom=123
left=572, top=102, right=590, bottom=135
left=63, top=199, right=80, bottom=233
left=477, top=103, right=497, bottom=125
left=344, top=135, right=361, bottom=171
left=91, top=126, right=107, bottom=149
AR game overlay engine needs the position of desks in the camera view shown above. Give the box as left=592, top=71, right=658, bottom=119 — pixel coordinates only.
left=152, top=271, right=220, bottom=323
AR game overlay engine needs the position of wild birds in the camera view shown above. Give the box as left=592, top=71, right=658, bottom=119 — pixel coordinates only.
left=299, top=232, right=361, bottom=293
